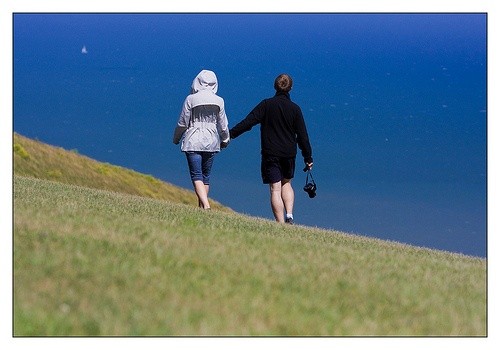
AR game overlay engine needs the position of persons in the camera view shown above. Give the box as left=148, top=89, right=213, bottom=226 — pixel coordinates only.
left=173, top=69, right=231, bottom=209
left=229, top=74, right=313, bottom=224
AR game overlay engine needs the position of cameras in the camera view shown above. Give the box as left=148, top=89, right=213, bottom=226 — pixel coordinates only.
left=304, top=183, right=316, bottom=198
